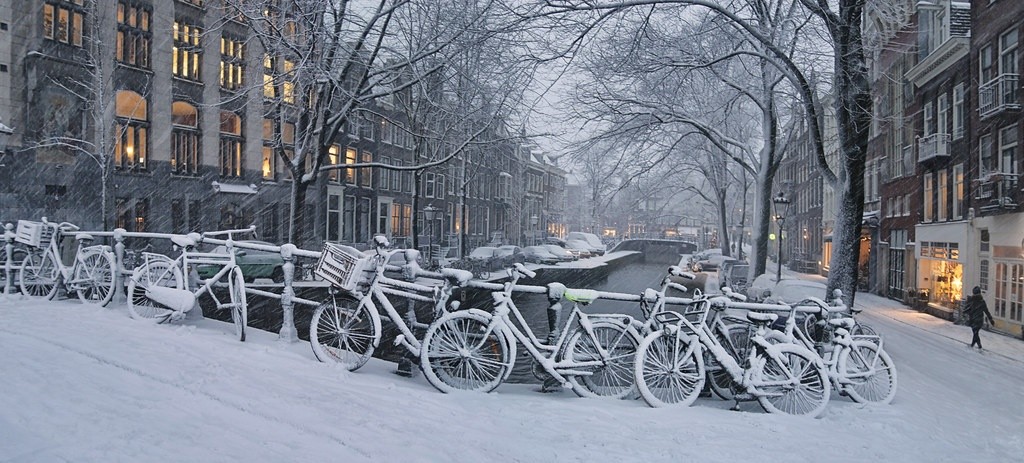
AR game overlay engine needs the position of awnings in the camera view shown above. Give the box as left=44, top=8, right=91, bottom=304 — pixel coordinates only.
left=211, top=180, right=260, bottom=196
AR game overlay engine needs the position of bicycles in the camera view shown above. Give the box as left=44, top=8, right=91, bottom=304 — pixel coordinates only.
left=126, top=223, right=257, bottom=341
left=308, top=234, right=518, bottom=395
left=292, top=255, right=325, bottom=282
left=427, top=253, right=492, bottom=282
left=420, top=260, right=648, bottom=401
left=14, top=215, right=118, bottom=307
left=632, top=263, right=898, bottom=419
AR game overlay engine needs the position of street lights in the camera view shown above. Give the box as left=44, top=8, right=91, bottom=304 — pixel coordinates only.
left=423, top=204, right=440, bottom=260
left=771, top=188, right=793, bottom=283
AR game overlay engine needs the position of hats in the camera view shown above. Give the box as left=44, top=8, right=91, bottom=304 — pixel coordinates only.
left=972, top=285, right=982, bottom=294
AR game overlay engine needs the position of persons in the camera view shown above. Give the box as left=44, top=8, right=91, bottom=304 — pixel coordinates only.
left=964, top=286, right=995, bottom=351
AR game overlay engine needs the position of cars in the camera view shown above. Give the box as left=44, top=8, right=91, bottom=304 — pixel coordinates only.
left=689, top=248, right=749, bottom=303
left=186, top=239, right=287, bottom=283
left=522, top=245, right=560, bottom=266
left=360, top=246, right=424, bottom=287
left=465, top=246, right=508, bottom=274
left=540, top=242, right=575, bottom=262
left=533, top=235, right=598, bottom=260
left=496, top=244, right=526, bottom=268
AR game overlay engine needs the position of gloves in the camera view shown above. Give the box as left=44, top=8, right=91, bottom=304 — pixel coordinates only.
left=990, top=319, right=995, bottom=327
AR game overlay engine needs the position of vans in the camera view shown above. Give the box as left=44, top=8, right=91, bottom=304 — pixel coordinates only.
left=568, top=230, right=606, bottom=256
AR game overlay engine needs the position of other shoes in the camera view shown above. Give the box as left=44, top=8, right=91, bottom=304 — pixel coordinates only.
left=968, top=343, right=975, bottom=350
left=977, top=347, right=984, bottom=353
